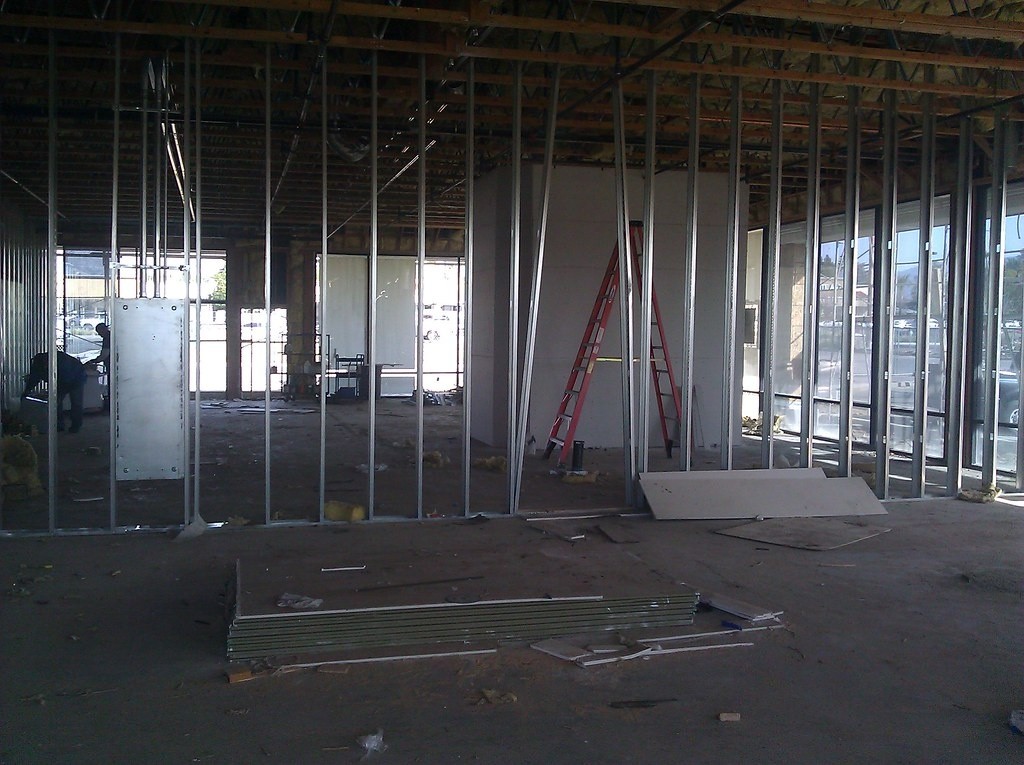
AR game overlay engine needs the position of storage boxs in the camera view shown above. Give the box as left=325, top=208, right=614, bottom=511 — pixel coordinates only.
left=335, top=387, right=355, bottom=398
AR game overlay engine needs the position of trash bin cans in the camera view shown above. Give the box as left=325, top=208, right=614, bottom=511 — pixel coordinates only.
left=361, top=364, right=382, bottom=400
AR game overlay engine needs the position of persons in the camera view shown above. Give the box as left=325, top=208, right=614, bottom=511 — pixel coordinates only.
left=20, top=351, right=86, bottom=433
left=82, top=323, right=110, bottom=377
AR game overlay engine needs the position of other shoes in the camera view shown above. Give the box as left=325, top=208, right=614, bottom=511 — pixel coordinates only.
left=57, top=424, right=65, bottom=432
left=69, top=420, right=83, bottom=433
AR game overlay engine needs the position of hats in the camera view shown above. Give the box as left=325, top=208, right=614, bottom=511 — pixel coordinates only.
left=96, top=323, right=110, bottom=331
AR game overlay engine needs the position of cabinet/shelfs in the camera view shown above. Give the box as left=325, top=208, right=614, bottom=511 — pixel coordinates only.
left=281, top=334, right=330, bottom=403
left=335, top=353, right=364, bottom=398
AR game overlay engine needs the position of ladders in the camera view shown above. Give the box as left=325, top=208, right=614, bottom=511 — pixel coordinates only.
left=544, top=221, right=694, bottom=469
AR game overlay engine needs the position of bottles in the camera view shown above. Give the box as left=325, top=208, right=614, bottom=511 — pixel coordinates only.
left=530, top=434, right=538, bottom=456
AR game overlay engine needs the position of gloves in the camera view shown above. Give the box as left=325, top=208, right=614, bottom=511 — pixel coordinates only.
left=20, top=388, right=29, bottom=400
